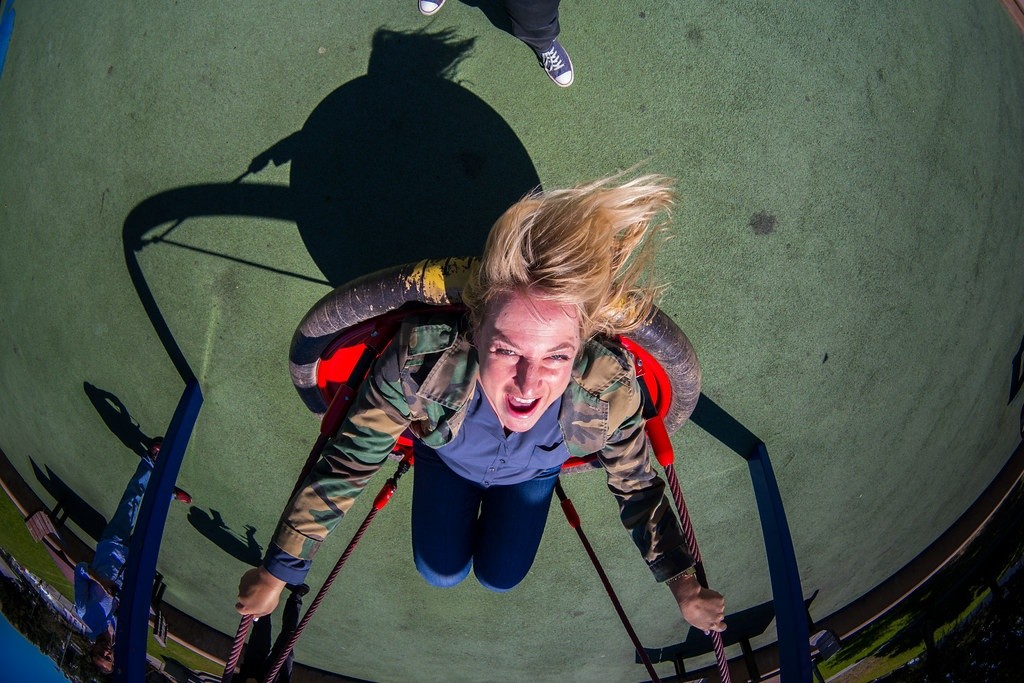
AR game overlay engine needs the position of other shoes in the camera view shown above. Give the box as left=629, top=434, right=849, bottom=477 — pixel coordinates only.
left=147, top=437, right=192, bottom=504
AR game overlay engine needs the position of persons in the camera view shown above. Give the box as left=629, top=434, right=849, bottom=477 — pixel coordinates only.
left=232, top=188, right=730, bottom=636
left=418, top=0, right=576, bottom=89
left=74, top=434, right=191, bottom=683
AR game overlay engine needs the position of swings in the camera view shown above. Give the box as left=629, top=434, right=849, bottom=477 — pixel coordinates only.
left=220, top=255, right=733, bottom=683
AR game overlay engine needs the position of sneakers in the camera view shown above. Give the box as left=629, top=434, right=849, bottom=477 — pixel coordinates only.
left=417, top=0, right=449, bottom=15
left=293, top=585, right=309, bottom=597
left=537, top=39, right=576, bottom=88
